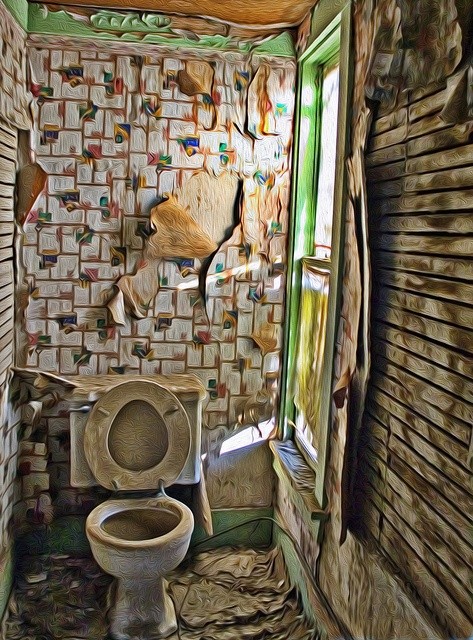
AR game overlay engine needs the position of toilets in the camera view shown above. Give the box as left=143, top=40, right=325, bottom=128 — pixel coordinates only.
left=68, top=374, right=208, bottom=640
